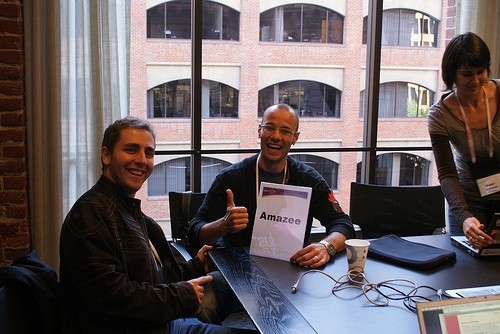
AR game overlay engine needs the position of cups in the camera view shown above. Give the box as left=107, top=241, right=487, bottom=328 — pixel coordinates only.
left=344, top=239, right=371, bottom=271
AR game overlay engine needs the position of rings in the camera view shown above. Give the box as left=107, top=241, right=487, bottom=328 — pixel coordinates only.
left=469, top=237, right=472, bottom=241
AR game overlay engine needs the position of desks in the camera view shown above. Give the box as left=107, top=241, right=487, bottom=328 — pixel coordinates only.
left=203, top=235, right=500, bottom=334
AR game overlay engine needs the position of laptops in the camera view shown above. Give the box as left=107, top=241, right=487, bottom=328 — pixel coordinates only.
left=450, top=213, right=500, bottom=258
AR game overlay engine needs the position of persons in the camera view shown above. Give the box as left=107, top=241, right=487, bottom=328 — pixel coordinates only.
left=188, top=103, right=356, bottom=326
left=427, top=32, right=500, bottom=248
left=59, top=115, right=259, bottom=334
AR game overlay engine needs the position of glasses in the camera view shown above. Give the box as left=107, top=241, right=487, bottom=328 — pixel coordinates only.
left=260, top=124, right=298, bottom=137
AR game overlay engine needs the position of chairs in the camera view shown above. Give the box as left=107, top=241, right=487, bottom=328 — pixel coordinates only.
left=0, top=249, right=63, bottom=334
left=350, top=181, right=446, bottom=239
left=169, top=191, right=207, bottom=243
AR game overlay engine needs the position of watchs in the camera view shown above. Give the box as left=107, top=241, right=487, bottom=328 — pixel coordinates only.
left=319, top=240, right=336, bottom=259
